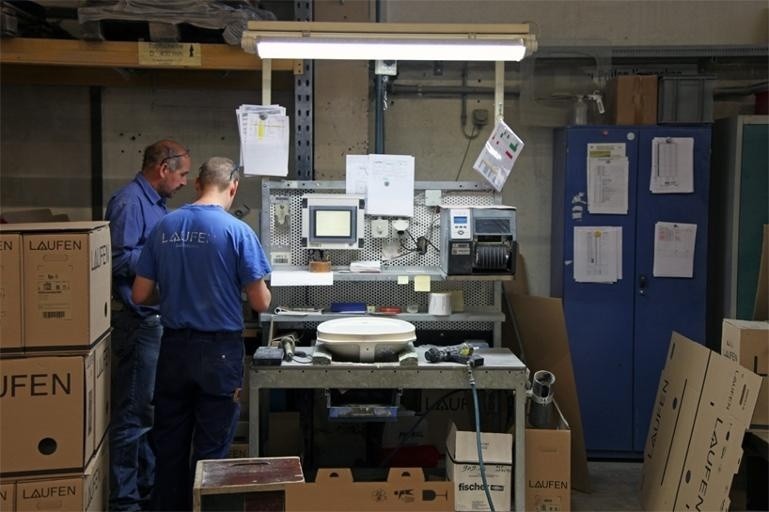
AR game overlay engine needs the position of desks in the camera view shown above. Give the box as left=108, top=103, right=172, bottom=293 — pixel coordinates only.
left=247, top=347, right=528, bottom=510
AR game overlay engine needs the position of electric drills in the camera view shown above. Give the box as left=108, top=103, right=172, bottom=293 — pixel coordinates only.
left=425, top=342, right=484, bottom=366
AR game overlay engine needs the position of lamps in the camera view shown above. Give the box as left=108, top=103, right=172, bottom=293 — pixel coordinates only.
left=244, top=18, right=539, bottom=62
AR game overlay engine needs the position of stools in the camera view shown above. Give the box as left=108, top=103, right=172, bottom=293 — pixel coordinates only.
left=193, top=455, right=306, bottom=511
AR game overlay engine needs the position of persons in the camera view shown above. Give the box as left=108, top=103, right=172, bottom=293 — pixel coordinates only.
left=131, top=155, right=274, bottom=511
left=103, top=138, right=192, bottom=512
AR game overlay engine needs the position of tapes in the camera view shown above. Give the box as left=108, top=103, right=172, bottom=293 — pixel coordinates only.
left=309, top=260, right=331, bottom=272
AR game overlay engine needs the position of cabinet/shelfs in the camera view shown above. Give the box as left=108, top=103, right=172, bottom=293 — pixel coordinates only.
left=259, top=268, right=516, bottom=324
left=549, top=126, right=711, bottom=464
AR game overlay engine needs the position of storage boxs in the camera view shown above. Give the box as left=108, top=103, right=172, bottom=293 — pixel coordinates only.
left=603, top=73, right=659, bottom=127
left=439, top=397, right=572, bottom=511
left=0, top=220, right=113, bottom=512
left=719, top=318, right=769, bottom=430
